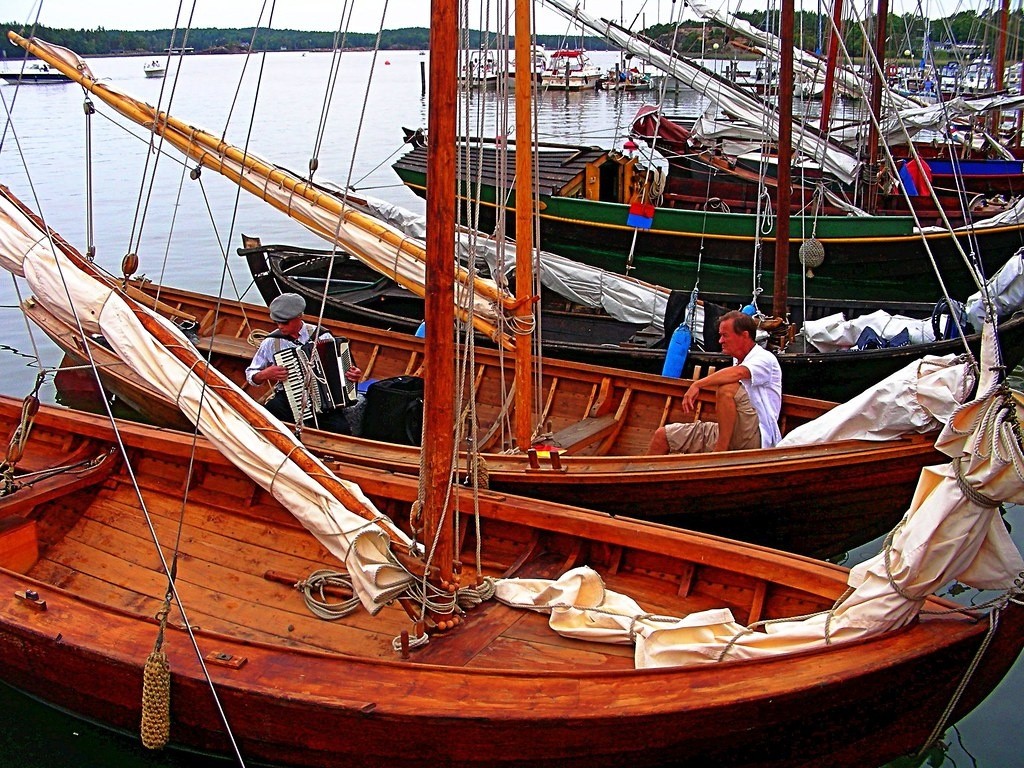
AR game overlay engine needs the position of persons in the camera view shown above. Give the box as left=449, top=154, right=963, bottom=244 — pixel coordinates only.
left=486, top=59, right=493, bottom=72
left=152, top=60, right=160, bottom=67
left=646, top=310, right=782, bottom=458
left=630, top=67, right=650, bottom=84
left=755, top=68, right=762, bottom=81
left=924, top=74, right=934, bottom=92
left=919, top=82, right=924, bottom=92
left=245, top=293, right=360, bottom=435
left=473, top=58, right=479, bottom=67
left=42, top=64, right=48, bottom=72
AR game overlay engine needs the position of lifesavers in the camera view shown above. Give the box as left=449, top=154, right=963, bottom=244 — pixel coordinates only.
left=887, top=65, right=897, bottom=76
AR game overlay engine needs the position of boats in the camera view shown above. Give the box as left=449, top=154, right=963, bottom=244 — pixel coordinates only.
left=457, top=50, right=499, bottom=87
left=602, top=67, right=657, bottom=91
left=720, top=69, right=751, bottom=76
left=542, top=49, right=604, bottom=91
left=143, top=63, right=165, bottom=77
left=498, top=43, right=548, bottom=91
left=0, top=71, right=76, bottom=86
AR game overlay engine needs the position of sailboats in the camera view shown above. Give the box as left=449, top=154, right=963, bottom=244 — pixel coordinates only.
left=0, top=23, right=1024, bottom=768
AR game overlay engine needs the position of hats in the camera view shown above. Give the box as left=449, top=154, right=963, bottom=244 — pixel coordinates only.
left=269, top=293, right=306, bottom=323
